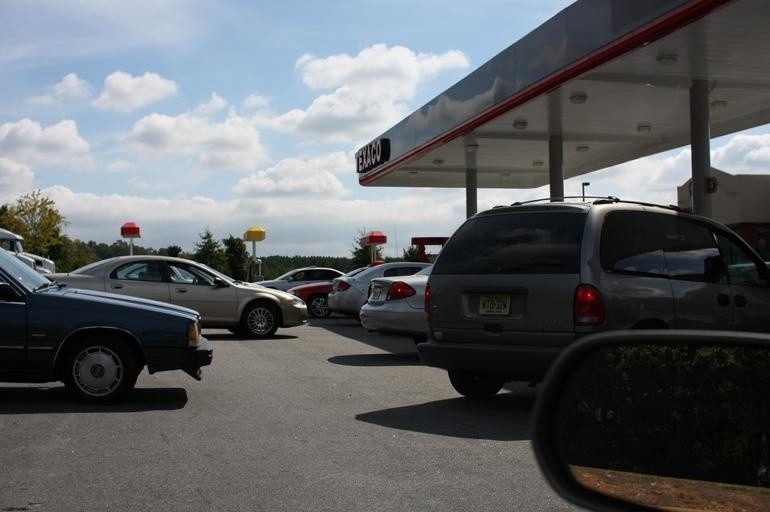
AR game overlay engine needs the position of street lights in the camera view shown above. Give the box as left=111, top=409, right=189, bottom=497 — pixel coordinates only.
left=582, top=182, right=591, bottom=203
